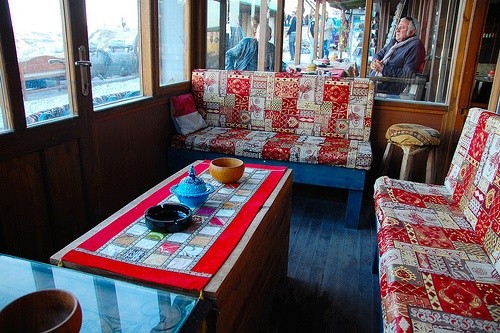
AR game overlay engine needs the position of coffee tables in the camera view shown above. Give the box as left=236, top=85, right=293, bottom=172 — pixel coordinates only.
left=50, top=160, right=293, bottom=333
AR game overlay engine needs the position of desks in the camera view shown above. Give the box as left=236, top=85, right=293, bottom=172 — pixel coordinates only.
left=0, top=252, right=202, bottom=333
left=291, top=60, right=359, bottom=79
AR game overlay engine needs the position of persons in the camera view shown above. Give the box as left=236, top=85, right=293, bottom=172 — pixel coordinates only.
left=368, top=17, right=426, bottom=96
left=225, top=25, right=287, bottom=71
left=287, top=11, right=296, bottom=60
left=308, top=13, right=321, bottom=59
left=319, top=11, right=334, bottom=59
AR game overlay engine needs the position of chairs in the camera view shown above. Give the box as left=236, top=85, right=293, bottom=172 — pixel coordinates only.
left=370, top=56, right=430, bottom=101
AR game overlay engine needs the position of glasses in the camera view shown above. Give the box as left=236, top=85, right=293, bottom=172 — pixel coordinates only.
left=406, top=16, right=415, bottom=27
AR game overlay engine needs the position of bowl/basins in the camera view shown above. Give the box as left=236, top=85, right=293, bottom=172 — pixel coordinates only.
left=168, top=165, right=215, bottom=206
left=0, top=287, right=83, bottom=333
left=209, top=157, right=245, bottom=184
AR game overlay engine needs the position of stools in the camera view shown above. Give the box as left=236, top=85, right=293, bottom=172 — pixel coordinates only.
left=377, top=123, right=441, bottom=184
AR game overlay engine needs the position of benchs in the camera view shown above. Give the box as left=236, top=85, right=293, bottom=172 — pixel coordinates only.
left=372, top=106, right=500, bottom=333
left=171, top=68, right=374, bottom=229
